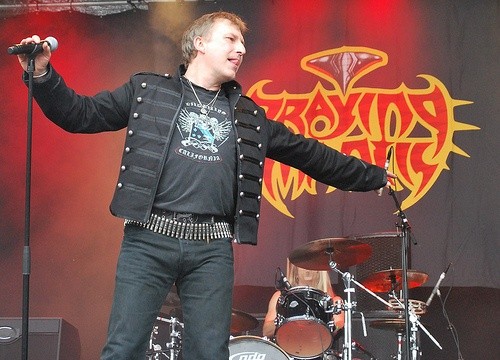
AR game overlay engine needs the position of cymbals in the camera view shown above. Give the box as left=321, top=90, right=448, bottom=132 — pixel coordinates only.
left=385, top=299, right=429, bottom=314
left=361, top=269, right=429, bottom=292
left=287, top=238, right=371, bottom=270
left=369, top=319, right=430, bottom=331
left=230, top=308, right=259, bottom=332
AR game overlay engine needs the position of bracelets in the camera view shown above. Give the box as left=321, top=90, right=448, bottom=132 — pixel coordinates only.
left=33, top=70, right=49, bottom=79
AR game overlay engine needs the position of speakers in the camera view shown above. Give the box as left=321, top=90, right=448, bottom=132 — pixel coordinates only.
left=0, top=316, right=82, bottom=360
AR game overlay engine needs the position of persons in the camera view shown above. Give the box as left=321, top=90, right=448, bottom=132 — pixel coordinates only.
left=263, top=264, right=344, bottom=338
left=18, top=12, right=397, bottom=360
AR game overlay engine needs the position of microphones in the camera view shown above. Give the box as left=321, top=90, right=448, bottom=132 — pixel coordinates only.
left=8, top=36, right=58, bottom=54
left=377, top=148, right=391, bottom=197
left=425, top=263, right=451, bottom=306
left=279, top=269, right=292, bottom=290
left=351, top=338, right=374, bottom=359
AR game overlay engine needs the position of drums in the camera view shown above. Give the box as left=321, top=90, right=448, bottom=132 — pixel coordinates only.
left=274, top=285, right=336, bottom=360
left=228, top=334, right=291, bottom=360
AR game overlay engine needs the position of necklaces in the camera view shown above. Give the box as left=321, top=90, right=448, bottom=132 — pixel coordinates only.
left=183, top=73, right=222, bottom=114
